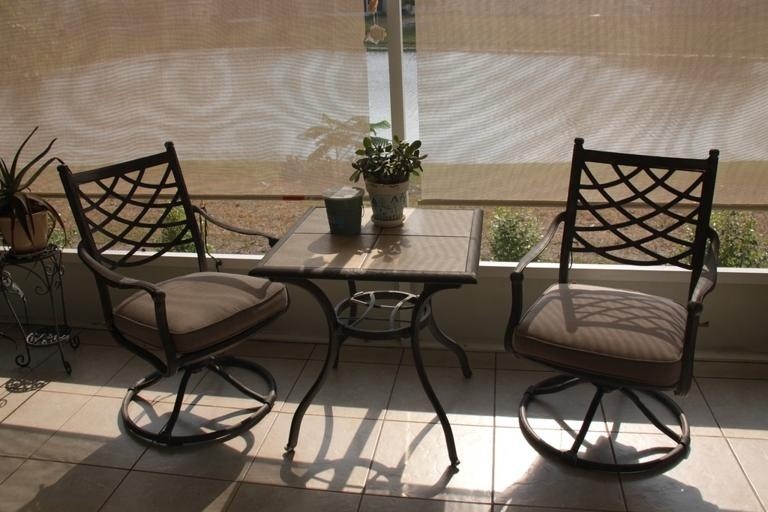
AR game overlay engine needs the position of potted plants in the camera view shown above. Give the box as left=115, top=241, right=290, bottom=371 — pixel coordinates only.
left=1, top=126, right=67, bottom=256
left=349, top=135, right=427, bottom=228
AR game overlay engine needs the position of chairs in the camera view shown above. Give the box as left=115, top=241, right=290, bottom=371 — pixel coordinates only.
left=56, top=142, right=292, bottom=454
left=503, top=136, right=721, bottom=477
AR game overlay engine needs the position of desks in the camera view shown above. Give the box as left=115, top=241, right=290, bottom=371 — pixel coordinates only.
left=249, top=204, right=484, bottom=471
left=2, top=245, right=81, bottom=377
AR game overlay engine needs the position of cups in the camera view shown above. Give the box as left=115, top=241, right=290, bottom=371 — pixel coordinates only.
left=324, top=186, right=364, bottom=234
